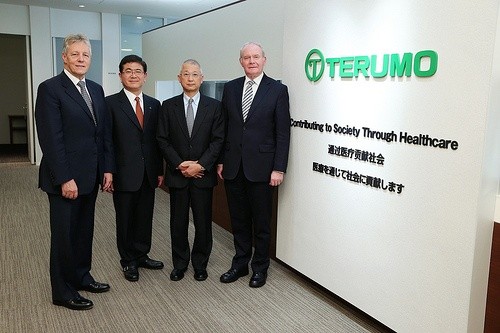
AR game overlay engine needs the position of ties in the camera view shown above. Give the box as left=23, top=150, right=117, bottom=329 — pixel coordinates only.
left=186, top=99, right=196, bottom=137
left=135, top=96, right=144, bottom=129
left=242, top=81, right=255, bottom=123
left=77, top=80, right=98, bottom=127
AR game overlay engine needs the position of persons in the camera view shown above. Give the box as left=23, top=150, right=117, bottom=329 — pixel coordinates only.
left=217, top=41, right=290, bottom=288
left=35, top=34, right=112, bottom=311
left=105, top=55, right=164, bottom=282
left=156, top=59, right=223, bottom=281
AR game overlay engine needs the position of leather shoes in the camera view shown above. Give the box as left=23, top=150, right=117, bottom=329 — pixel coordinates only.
left=249, top=270, right=267, bottom=288
left=139, top=255, right=164, bottom=270
left=193, top=266, right=208, bottom=281
left=120, top=257, right=140, bottom=282
left=78, top=280, right=110, bottom=294
left=170, top=266, right=187, bottom=281
left=52, top=293, right=94, bottom=311
left=219, top=267, right=249, bottom=283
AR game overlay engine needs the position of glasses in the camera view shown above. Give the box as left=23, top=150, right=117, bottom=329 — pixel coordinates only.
left=121, top=70, right=144, bottom=76
left=180, top=73, right=199, bottom=79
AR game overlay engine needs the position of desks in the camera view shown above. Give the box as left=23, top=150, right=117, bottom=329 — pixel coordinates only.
left=8, top=115, right=27, bottom=144
